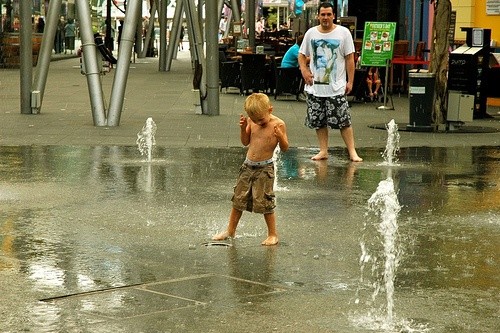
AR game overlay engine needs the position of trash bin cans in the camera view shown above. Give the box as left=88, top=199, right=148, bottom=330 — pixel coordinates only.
left=408, top=69, right=436, bottom=125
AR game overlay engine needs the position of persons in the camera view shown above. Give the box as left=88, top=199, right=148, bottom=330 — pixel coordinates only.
left=212, top=93, right=289, bottom=246
left=0, top=10, right=382, bottom=98
left=298, top=3, right=363, bottom=162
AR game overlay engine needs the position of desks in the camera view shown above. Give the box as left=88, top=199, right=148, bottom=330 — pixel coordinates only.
left=266, top=56, right=281, bottom=63
left=230, top=48, right=254, bottom=62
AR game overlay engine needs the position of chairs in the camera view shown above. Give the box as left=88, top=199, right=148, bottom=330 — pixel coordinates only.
left=201, top=30, right=428, bottom=104
